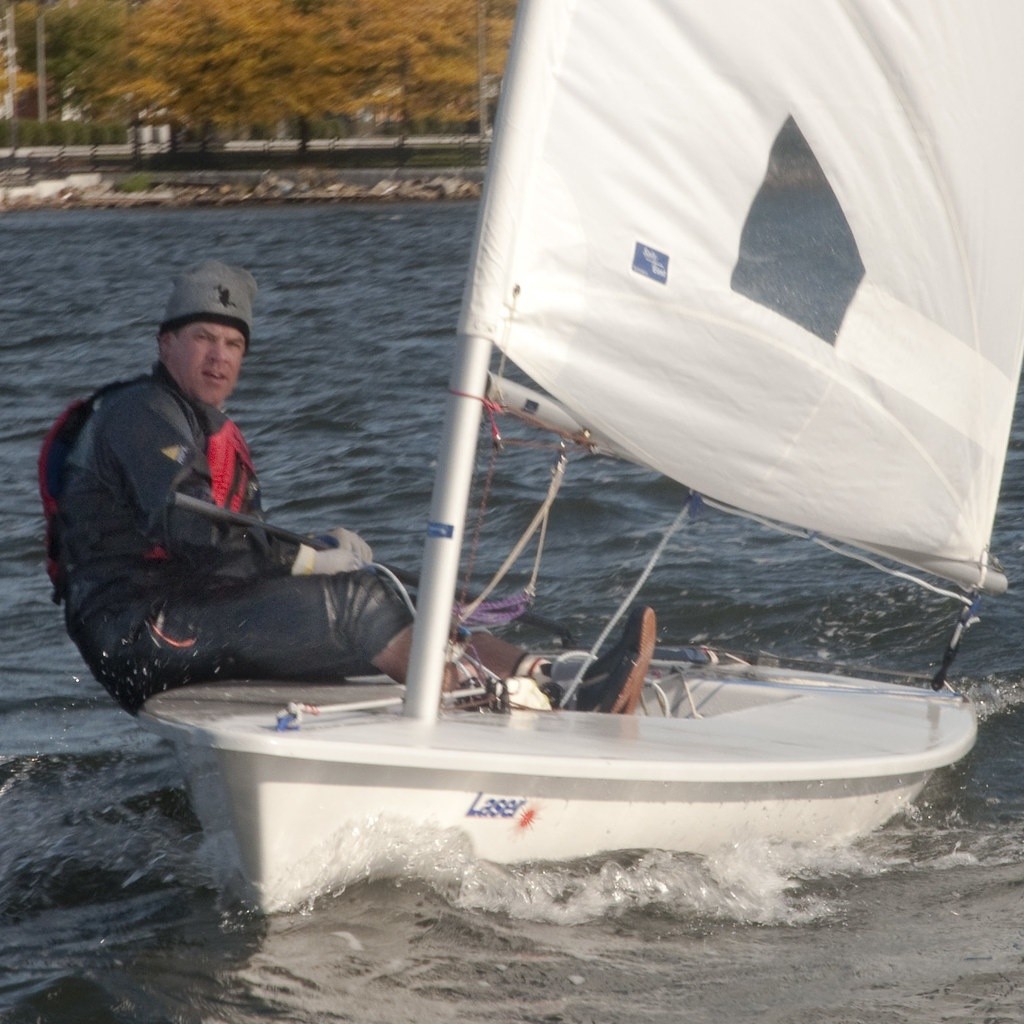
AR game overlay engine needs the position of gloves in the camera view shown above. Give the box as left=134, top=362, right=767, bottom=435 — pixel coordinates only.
left=287, top=524, right=374, bottom=578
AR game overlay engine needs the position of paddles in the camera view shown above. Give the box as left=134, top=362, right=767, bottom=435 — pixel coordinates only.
left=164, top=490, right=574, bottom=638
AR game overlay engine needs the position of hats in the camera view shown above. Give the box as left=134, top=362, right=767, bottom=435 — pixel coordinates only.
left=161, top=259, right=256, bottom=349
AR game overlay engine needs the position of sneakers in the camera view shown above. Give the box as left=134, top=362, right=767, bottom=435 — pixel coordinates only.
left=540, top=607, right=658, bottom=715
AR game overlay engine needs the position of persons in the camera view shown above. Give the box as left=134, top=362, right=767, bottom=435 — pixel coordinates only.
left=37, top=257, right=655, bottom=715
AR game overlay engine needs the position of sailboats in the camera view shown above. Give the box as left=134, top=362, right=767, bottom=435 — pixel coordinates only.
left=138, top=0, right=1022, bottom=917
